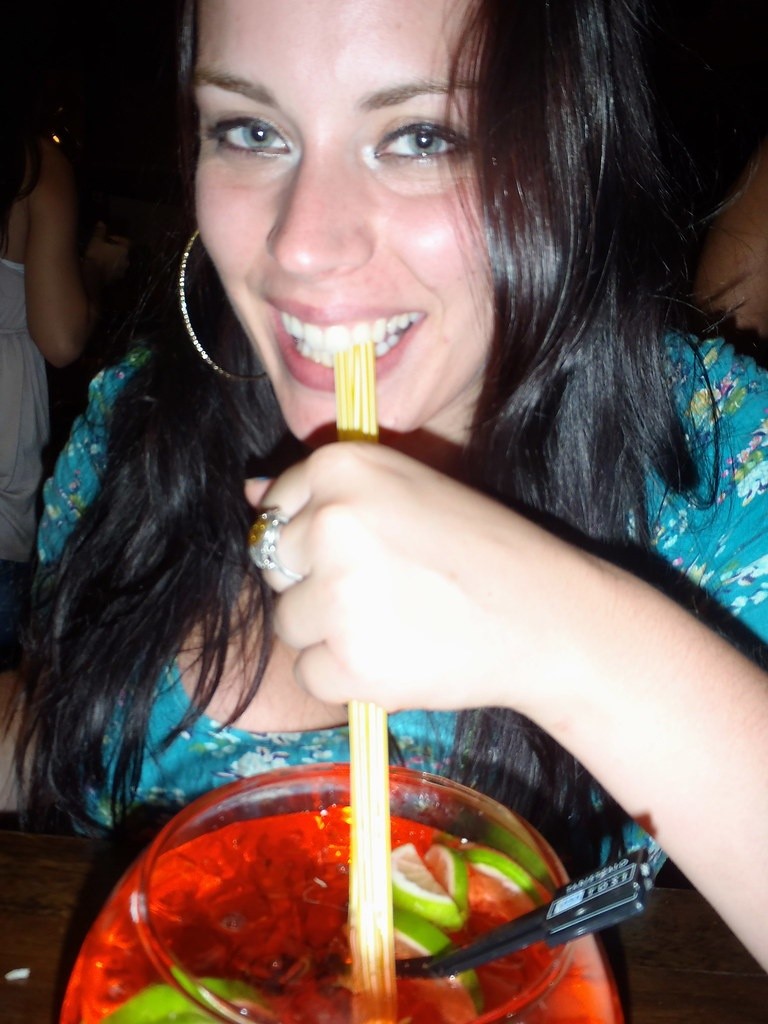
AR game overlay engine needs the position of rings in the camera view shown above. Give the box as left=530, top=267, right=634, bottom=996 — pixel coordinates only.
left=247, top=512, right=302, bottom=585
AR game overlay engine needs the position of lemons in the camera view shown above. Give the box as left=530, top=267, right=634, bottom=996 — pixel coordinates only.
left=94, top=838, right=551, bottom=1024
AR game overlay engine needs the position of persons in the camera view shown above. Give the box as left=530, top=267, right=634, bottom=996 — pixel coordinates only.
left=1, top=1, right=768, bottom=977
left=2, top=49, right=127, bottom=729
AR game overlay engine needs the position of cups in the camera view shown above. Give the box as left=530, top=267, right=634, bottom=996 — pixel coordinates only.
left=56, top=763, right=626, bottom=1024
